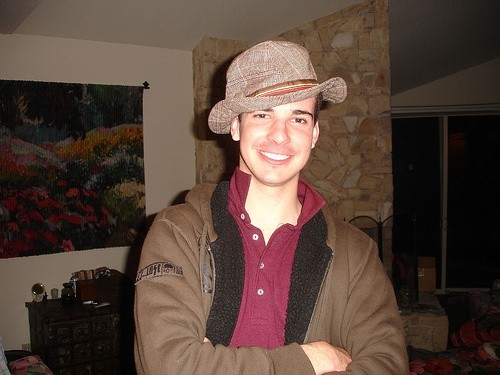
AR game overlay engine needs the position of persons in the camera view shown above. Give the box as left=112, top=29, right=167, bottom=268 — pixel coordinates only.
left=133, top=40, right=408, bottom=375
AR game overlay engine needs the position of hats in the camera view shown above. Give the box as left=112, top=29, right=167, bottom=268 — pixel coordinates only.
left=207, top=41, right=348, bottom=134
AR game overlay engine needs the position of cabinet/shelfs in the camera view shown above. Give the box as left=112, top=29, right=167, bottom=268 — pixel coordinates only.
left=26, top=298, right=120, bottom=375
